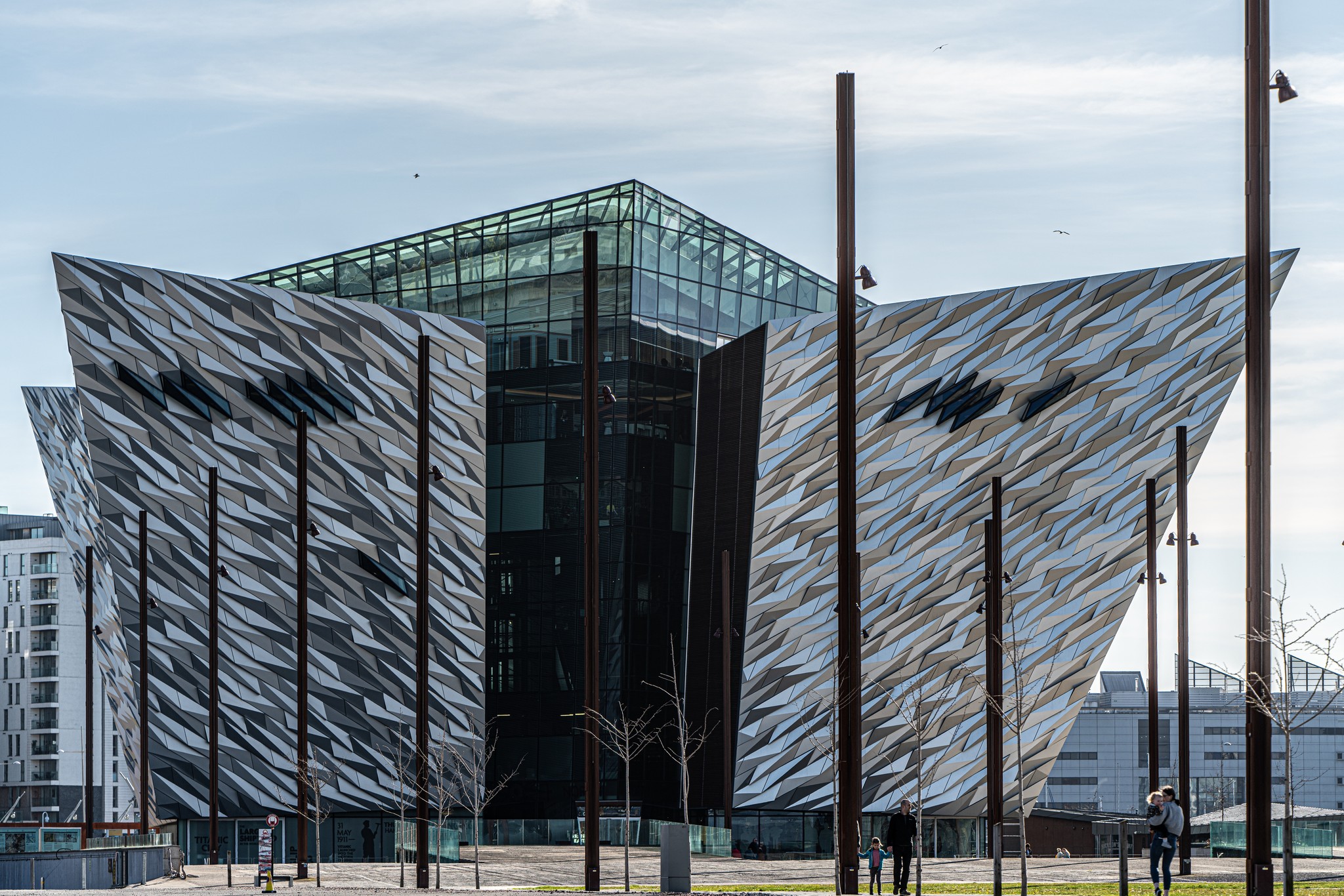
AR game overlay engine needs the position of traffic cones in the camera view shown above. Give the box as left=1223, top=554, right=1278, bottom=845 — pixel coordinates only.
left=262, top=870, right=277, bottom=893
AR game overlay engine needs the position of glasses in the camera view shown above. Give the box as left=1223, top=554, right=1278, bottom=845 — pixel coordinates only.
left=1162, top=794, right=1169, bottom=797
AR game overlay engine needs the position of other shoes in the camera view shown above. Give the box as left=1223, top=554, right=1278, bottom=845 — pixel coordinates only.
left=878, top=893, right=882, bottom=896
left=870, top=892, right=873, bottom=895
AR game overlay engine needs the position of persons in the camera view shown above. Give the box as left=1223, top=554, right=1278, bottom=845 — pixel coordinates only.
left=760, top=841, right=767, bottom=861
left=743, top=847, right=756, bottom=860
left=732, top=848, right=741, bottom=858
left=1025, top=843, right=1032, bottom=858
left=748, top=838, right=762, bottom=860
left=857, top=837, right=892, bottom=896
left=1057, top=848, right=1062, bottom=858
left=1062, top=848, right=1070, bottom=858
left=1146, top=791, right=1172, bottom=848
left=1147, top=785, right=1184, bottom=896
left=837, top=820, right=861, bottom=870
left=361, top=820, right=378, bottom=863
left=887, top=799, right=918, bottom=896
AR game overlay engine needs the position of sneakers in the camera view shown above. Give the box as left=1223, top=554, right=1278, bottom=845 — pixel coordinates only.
left=900, top=890, right=911, bottom=896
left=1155, top=887, right=1162, bottom=896
left=893, top=888, right=899, bottom=895
left=1162, top=842, right=1172, bottom=848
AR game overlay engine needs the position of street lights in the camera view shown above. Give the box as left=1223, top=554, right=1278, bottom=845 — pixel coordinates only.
left=57, top=726, right=85, bottom=823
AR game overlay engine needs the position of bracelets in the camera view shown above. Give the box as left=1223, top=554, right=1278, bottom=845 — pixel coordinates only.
left=916, top=843, right=917, bottom=844
